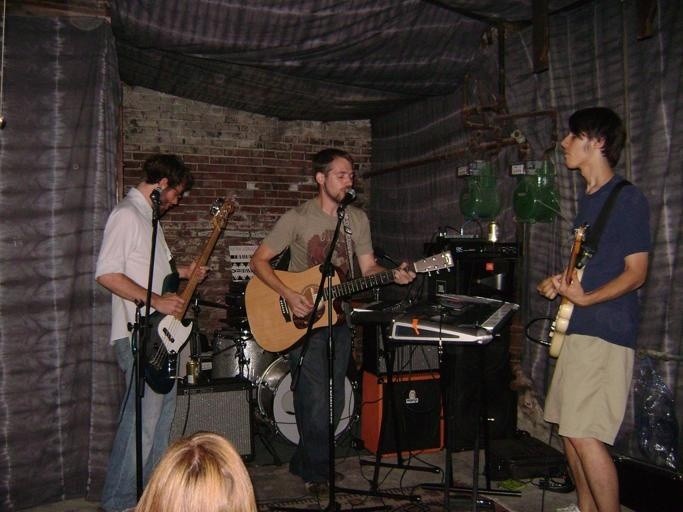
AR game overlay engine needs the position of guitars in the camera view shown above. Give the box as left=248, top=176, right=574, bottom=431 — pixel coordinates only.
left=131, top=197, right=234, bottom=394
left=245, top=251, right=455, bottom=352
left=548, top=221, right=587, bottom=358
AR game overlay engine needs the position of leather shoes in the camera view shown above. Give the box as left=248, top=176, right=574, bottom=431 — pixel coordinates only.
left=325, top=471, right=344, bottom=482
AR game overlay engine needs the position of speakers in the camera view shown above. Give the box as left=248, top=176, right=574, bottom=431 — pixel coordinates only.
left=425, top=241, right=516, bottom=323
left=166, top=376, right=253, bottom=467
left=361, top=371, right=445, bottom=457
left=444, top=339, right=518, bottom=451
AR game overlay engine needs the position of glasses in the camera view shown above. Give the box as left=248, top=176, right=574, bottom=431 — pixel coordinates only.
left=172, top=187, right=182, bottom=199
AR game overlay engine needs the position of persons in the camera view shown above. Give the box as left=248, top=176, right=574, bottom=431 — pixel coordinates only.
left=93, top=151, right=208, bottom=510
left=135, top=429, right=258, bottom=510
left=540, top=104, right=651, bottom=511
left=250, top=146, right=415, bottom=484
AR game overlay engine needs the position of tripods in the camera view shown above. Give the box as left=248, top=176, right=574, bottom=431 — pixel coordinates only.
left=269, top=271, right=395, bottom=512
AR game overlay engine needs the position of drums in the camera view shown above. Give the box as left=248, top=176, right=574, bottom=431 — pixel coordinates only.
left=210, top=328, right=273, bottom=387
left=256, top=354, right=354, bottom=448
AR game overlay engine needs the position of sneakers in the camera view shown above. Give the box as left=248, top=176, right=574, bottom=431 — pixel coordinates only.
left=556, top=504, right=581, bottom=512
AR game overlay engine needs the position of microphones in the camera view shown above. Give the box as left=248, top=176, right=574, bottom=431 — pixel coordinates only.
left=150, top=186, right=162, bottom=200
left=336, top=189, right=355, bottom=214
